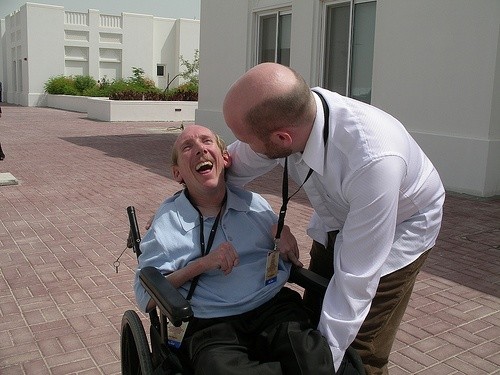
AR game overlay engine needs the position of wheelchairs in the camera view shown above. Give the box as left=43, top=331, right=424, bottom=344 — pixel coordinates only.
left=121, top=205, right=330, bottom=374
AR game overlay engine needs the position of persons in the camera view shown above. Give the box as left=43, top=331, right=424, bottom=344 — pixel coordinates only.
left=134, top=123, right=336, bottom=375
left=144, top=60, right=445, bottom=374
